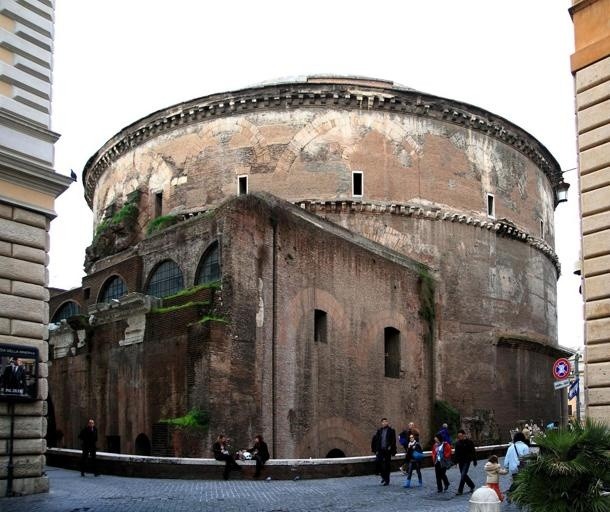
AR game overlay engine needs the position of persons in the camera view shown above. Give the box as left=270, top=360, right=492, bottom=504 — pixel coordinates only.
left=77, top=418, right=104, bottom=477
left=502, top=431, right=531, bottom=492
left=212, top=436, right=242, bottom=479
left=3, top=357, right=27, bottom=395
left=484, top=455, right=507, bottom=502
left=374, top=417, right=479, bottom=494
left=245, top=435, right=270, bottom=478
left=521, top=423, right=530, bottom=441
left=532, top=423, right=542, bottom=435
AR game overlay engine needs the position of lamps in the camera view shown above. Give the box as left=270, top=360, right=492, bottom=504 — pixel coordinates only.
left=554, top=165, right=579, bottom=202
left=573, top=250, right=582, bottom=275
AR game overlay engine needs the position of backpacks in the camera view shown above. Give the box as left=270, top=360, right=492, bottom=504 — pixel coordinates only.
left=371, top=435, right=377, bottom=448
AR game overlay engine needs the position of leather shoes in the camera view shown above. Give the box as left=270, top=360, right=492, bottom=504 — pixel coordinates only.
left=437, top=487, right=444, bottom=493
left=381, top=480, right=389, bottom=486
left=445, top=482, right=449, bottom=491
left=456, top=491, right=464, bottom=495
left=470, top=484, right=475, bottom=493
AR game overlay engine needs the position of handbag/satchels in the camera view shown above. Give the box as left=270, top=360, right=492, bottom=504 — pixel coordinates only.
left=413, top=451, right=424, bottom=459
left=518, top=461, right=526, bottom=469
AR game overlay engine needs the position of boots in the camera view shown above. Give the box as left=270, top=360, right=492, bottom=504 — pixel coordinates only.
left=417, top=479, right=422, bottom=487
left=403, top=479, right=411, bottom=487
